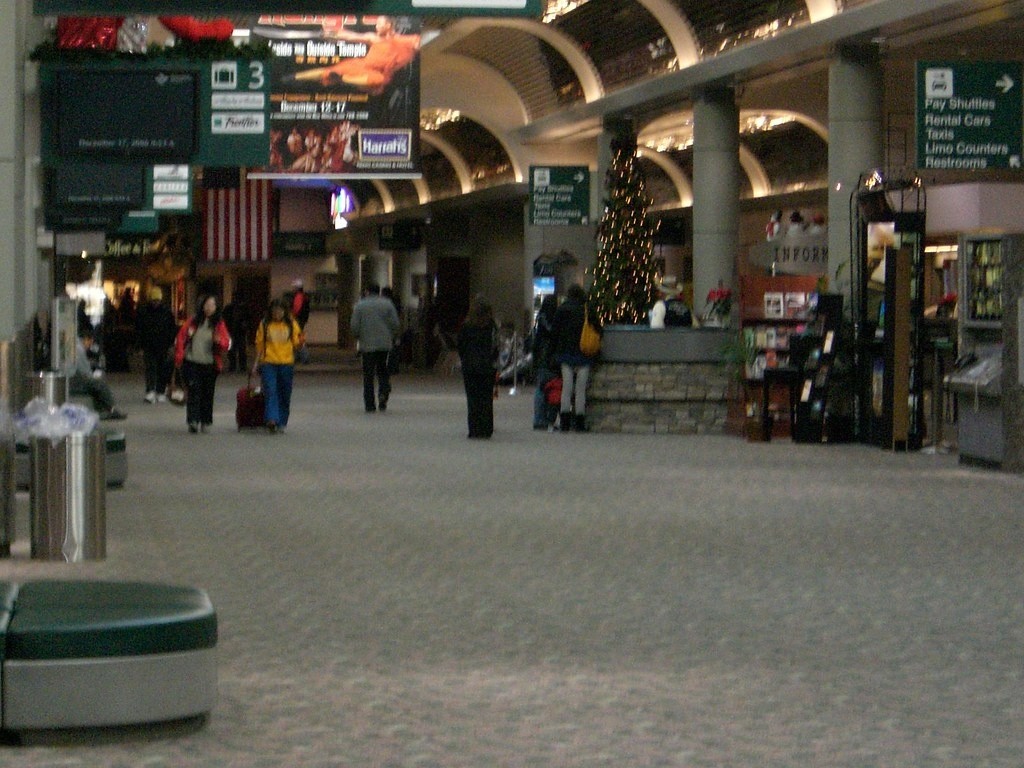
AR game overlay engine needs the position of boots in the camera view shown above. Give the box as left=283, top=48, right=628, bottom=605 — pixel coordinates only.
left=559, top=411, right=571, bottom=432
left=577, top=414, right=586, bottom=434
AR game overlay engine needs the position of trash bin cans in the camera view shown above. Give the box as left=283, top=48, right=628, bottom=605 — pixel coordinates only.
left=23, top=430, right=108, bottom=561
left=30, top=369, right=74, bottom=412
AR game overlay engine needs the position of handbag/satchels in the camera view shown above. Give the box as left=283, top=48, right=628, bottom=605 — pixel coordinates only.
left=579, top=302, right=600, bottom=356
left=544, top=376, right=564, bottom=404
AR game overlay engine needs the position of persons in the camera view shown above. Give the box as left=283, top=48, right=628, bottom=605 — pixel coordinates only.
left=134, top=288, right=177, bottom=404
left=381, top=285, right=405, bottom=374
left=458, top=293, right=500, bottom=439
left=281, top=280, right=310, bottom=331
left=534, top=284, right=605, bottom=433
left=651, top=275, right=700, bottom=330
left=271, top=120, right=360, bottom=173
left=223, top=294, right=250, bottom=375
left=66, top=331, right=128, bottom=422
left=251, top=300, right=304, bottom=436
left=174, top=294, right=232, bottom=434
left=77, top=285, right=141, bottom=372
left=350, top=280, right=401, bottom=413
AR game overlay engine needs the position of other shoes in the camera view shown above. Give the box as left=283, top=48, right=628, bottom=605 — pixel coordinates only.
left=202, top=424, right=214, bottom=433
left=188, top=418, right=199, bottom=432
left=156, top=394, right=165, bottom=402
left=534, top=419, right=560, bottom=431
left=145, top=390, right=155, bottom=403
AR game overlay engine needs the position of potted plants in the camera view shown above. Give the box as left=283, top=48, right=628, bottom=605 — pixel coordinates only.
left=707, top=328, right=771, bottom=443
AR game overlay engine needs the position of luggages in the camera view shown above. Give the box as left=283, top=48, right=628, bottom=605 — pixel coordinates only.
left=235, top=370, right=266, bottom=434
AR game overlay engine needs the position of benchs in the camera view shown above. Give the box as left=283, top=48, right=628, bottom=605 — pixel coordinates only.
left=0, top=580, right=218, bottom=732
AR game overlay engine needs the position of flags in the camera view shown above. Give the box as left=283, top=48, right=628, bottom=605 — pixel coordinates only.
left=200, top=166, right=273, bottom=262
